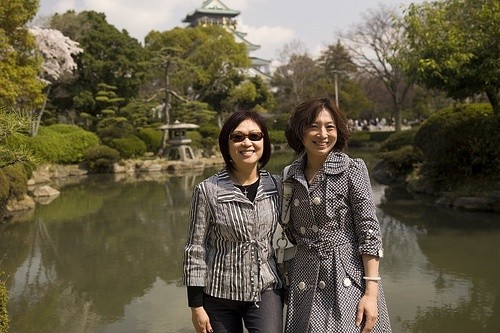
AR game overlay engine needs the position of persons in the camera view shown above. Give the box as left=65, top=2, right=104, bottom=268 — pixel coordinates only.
left=282, top=97, right=392, bottom=333
left=181, top=109, right=282, bottom=333
left=345, top=115, right=413, bottom=131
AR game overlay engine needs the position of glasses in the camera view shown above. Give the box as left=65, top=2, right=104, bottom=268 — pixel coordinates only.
left=228, top=131, right=265, bottom=142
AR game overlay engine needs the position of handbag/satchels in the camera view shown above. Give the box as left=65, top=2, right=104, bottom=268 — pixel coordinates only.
left=272, top=245, right=297, bottom=304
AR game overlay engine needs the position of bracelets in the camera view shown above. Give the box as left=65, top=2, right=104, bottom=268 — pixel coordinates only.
left=362, top=276, right=381, bottom=281
